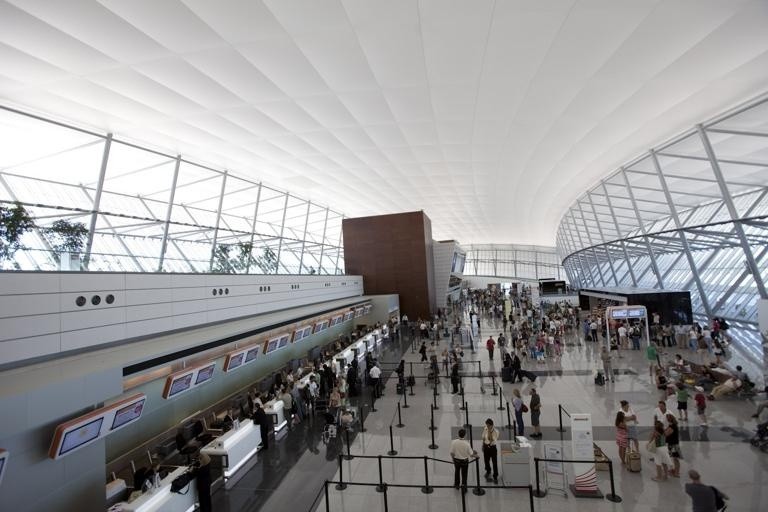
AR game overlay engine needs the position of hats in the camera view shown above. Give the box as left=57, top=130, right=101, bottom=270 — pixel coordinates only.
left=695, top=386, right=704, bottom=392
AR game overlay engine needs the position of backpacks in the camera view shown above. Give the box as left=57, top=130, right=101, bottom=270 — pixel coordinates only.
left=292, top=399, right=297, bottom=413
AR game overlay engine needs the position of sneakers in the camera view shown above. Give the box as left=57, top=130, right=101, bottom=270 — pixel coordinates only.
left=485, top=472, right=498, bottom=478
left=530, top=432, right=542, bottom=437
left=651, top=469, right=679, bottom=481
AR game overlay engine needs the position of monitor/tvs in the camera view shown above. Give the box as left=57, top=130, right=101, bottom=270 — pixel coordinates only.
left=363, top=305, right=372, bottom=316
left=312, top=321, right=329, bottom=334
left=224, top=345, right=260, bottom=374
left=0, top=448, right=9, bottom=482
left=263, top=333, right=289, bottom=356
left=48, top=393, right=147, bottom=462
left=162, top=360, right=216, bottom=400
left=292, top=326, right=312, bottom=344
left=354, top=308, right=365, bottom=319
left=329, top=315, right=343, bottom=328
left=343, top=312, right=354, bottom=323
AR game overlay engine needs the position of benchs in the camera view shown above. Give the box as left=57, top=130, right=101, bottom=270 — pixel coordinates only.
left=664, top=359, right=755, bottom=400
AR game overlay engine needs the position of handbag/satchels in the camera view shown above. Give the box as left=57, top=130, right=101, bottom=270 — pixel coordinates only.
left=712, top=487, right=726, bottom=512
left=521, top=404, right=528, bottom=412
left=170, top=471, right=192, bottom=492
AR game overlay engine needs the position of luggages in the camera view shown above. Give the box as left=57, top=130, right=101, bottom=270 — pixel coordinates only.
left=502, top=368, right=511, bottom=381
left=523, top=370, right=536, bottom=381
left=397, top=383, right=404, bottom=394
left=627, top=438, right=641, bottom=472
